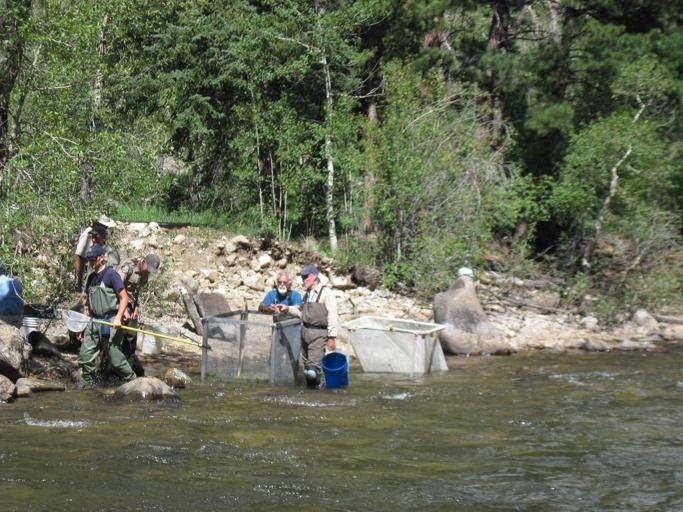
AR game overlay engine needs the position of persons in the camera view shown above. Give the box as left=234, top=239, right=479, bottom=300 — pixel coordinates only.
left=74, top=213, right=118, bottom=293
left=72, top=245, right=139, bottom=388
left=100, top=253, right=162, bottom=382
left=273, top=263, right=338, bottom=388
left=257, top=270, right=304, bottom=387
left=57, top=224, right=119, bottom=355
left=0, top=263, right=24, bottom=330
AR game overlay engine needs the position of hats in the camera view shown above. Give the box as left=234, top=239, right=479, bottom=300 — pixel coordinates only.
left=295, top=264, right=319, bottom=276
left=84, top=245, right=105, bottom=260
left=97, top=215, right=116, bottom=229
left=145, top=254, right=160, bottom=274
left=87, top=220, right=108, bottom=236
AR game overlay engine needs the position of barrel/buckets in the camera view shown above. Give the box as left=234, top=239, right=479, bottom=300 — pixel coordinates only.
left=0, top=274, right=22, bottom=314
left=22, top=316, right=39, bottom=336
left=321, top=353, right=348, bottom=387
